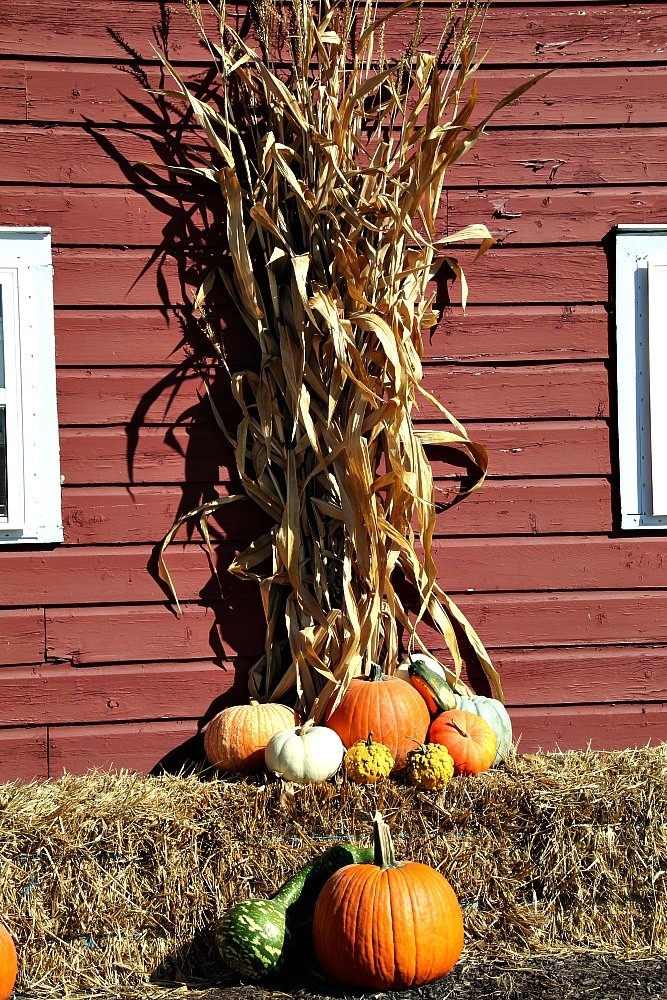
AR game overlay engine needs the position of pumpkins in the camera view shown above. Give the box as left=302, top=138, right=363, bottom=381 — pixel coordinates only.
left=0, top=924, right=18, bottom=1000
left=311, top=812, right=465, bottom=989
left=203, top=654, right=512, bottom=789
